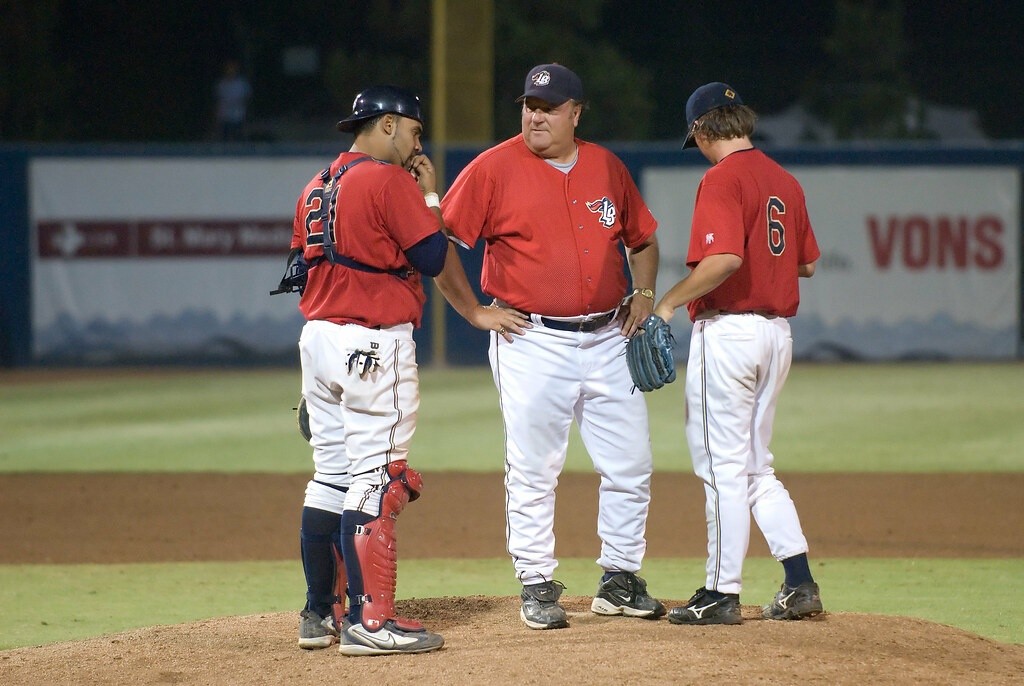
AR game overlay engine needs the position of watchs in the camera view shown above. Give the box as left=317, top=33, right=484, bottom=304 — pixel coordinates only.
left=633, top=288, right=655, bottom=300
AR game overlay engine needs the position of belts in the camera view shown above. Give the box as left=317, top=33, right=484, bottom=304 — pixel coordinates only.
left=370, top=324, right=380, bottom=330
left=511, top=308, right=616, bottom=332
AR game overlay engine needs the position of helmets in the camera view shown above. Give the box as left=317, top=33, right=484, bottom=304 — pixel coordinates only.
left=336, top=84, right=424, bottom=134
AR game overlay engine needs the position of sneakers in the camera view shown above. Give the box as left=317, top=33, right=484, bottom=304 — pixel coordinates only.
left=338, top=616, right=444, bottom=656
left=668, top=586, right=744, bottom=625
left=590, top=572, right=666, bottom=618
left=519, top=580, right=569, bottom=629
left=761, top=580, right=823, bottom=620
left=298, top=608, right=341, bottom=647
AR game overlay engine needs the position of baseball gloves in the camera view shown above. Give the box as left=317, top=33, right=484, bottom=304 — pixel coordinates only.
left=621, top=315, right=677, bottom=395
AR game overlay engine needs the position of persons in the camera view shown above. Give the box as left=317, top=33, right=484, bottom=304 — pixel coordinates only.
left=289, top=89, right=448, bottom=658
left=633, top=81, right=826, bottom=624
left=434, top=62, right=670, bottom=631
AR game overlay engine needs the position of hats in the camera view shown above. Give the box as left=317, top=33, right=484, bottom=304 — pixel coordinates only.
left=515, top=63, right=584, bottom=106
left=681, top=82, right=744, bottom=150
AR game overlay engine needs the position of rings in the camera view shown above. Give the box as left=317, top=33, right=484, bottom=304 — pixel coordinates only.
left=499, top=326, right=506, bottom=335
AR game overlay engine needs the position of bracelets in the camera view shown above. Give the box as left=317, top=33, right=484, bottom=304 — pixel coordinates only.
left=424, top=193, right=441, bottom=208
